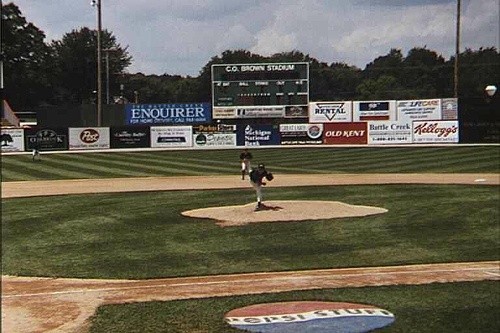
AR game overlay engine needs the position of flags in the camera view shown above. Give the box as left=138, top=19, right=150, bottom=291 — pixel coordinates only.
left=3, top=100, right=20, bottom=129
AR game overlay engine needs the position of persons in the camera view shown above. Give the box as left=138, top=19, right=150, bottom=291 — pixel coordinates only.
left=240, top=148, right=252, bottom=180
left=32, top=142, right=43, bottom=162
left=248, top=163, right=273, bottom=211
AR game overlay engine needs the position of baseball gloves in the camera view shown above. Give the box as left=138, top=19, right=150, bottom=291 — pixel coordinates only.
left=266, top=172, right=273, bottom=181
